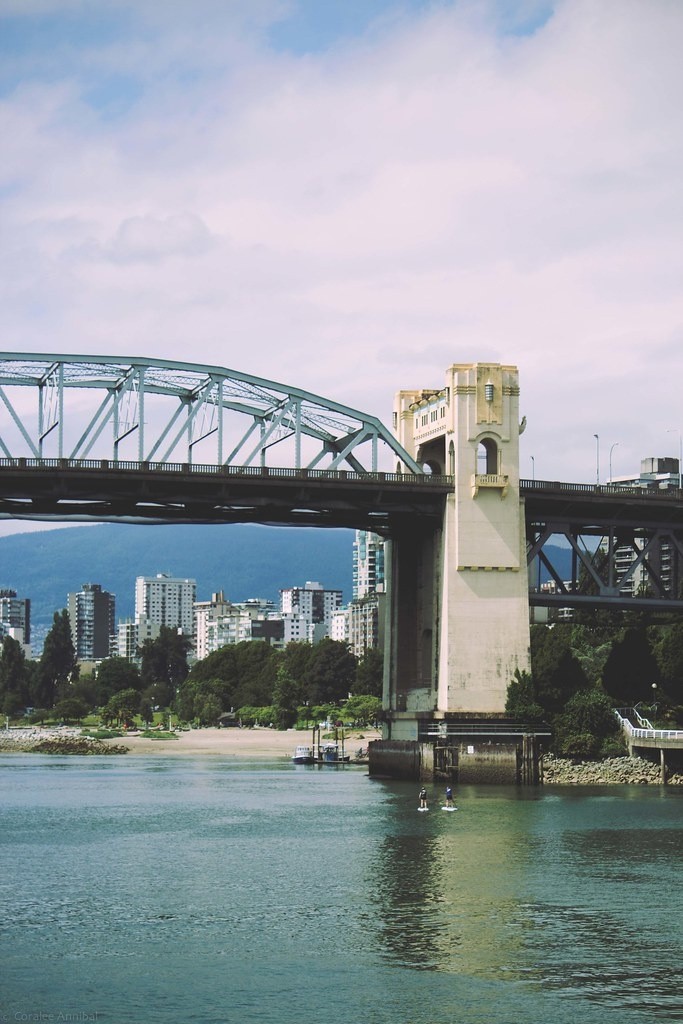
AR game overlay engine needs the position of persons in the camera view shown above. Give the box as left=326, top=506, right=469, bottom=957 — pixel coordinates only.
left=419, top=787, right=427, bottom=808
left=445, top=787, right=457, bottom=808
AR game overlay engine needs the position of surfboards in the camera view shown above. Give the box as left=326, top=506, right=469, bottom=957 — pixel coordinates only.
left=441, top=806, right=458, bottom=812
left=417, top=807, right=430, bottom=813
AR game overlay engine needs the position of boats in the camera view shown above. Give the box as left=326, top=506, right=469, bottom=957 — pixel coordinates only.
left=291, top=745, right=313, bottom=764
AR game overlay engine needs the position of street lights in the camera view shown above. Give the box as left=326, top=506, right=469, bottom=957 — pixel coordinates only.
left=530, top=456, right=534, bottom=479
left=609, top=442, right=617, bottom=486
left=594, top=434, right=599, bottom=485
left=667, top=429, right=682, bottom=488
left=652, top=683, right=658, bottom=704
